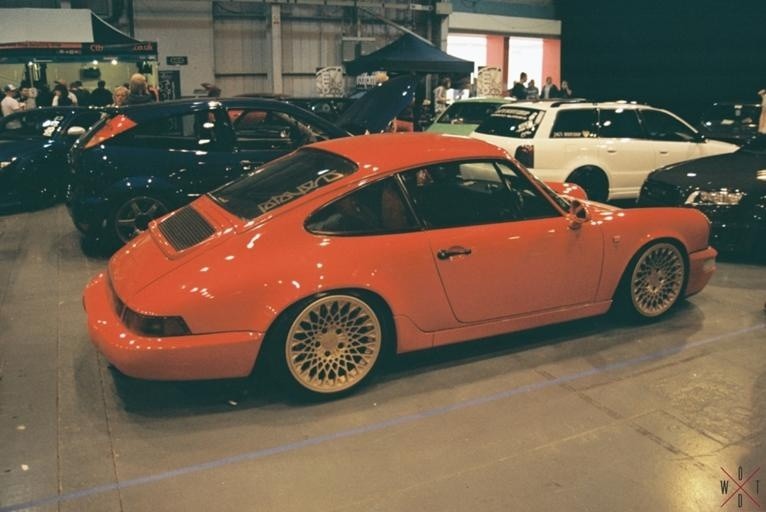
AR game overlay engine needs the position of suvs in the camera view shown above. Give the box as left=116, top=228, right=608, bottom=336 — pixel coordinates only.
left=473, top=97, right=740, bottom=209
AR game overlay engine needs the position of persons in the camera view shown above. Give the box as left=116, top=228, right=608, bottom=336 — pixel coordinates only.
left=510, top=72, right=572, bottom=100
left=1, top=73, right=160, bottom=130
left=194, top=83, right=237, bottom=142
left=433, top=78, right=451, bottom=119
left=469, top=78, right=477, bottom=98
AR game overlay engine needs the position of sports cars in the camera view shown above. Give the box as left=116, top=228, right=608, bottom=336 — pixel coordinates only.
left=79, top=131, right=721, bottom=405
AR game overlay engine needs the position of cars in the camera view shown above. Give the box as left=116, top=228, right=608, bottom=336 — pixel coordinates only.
left=276, top=95, right=368, bottom=126
left=66, top=74, right=425, bottom=255
left=208, top=92, right=288, bottom=128
left=637, top=135, right=766, bottom=266
left=426, top=98, right=520, bottom=140
left=700, top=102, right=762, bottom=144
left=0, top=105, right=95, bottom=216
